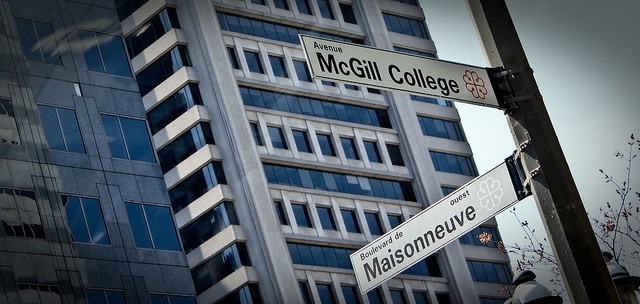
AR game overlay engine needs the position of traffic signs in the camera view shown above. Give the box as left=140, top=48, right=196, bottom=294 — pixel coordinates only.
left=300, top=35, right=500, bottom=107
left=350, top=159, right=519, bottom=295
